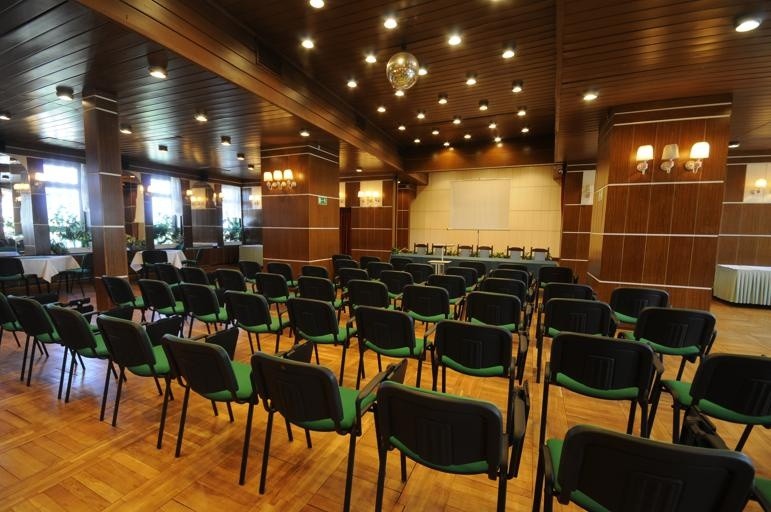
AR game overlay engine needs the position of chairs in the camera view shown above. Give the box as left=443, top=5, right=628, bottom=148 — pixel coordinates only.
left=142, top=248, right=204, bottom=280
left=0, top=250, right=93, bottom=298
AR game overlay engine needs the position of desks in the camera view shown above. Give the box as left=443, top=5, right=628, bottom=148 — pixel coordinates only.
left=135, top=250, right=183, bottom=279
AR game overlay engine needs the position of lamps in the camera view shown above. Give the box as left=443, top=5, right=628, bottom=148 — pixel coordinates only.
left=385, top=24, right=421, bottom=92
left=635, top=141, right=711, bottom=177
left=264, top=169, right=297, bottom=191
